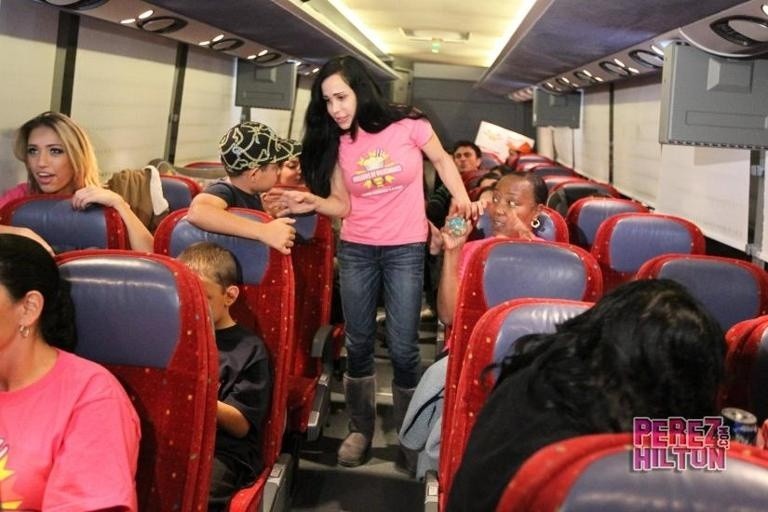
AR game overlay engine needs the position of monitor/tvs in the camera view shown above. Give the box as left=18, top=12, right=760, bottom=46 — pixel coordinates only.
left=234, top=61, right=296, bottom=110
left=532, top=88, right=581, bottom=128
left=656, top=41, right=768, bottom=151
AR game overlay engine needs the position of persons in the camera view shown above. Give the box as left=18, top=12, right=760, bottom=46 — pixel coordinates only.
left=1, top=230, right=144, bottom=512
left=443, top=273, right=730, bottom=512
left=172, top=243, right=274, bottom=509
left=0, top=110, right=552, bottom=361
left=260, top=54, right=487, bottom=470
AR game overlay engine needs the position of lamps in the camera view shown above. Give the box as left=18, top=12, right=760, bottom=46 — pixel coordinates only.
left=400, top=25, right=470, bottom=54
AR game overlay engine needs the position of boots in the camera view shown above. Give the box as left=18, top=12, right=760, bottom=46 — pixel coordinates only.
left=393, top=379, right=417, bottom=480
left=335, top=372, right=379, bottom=468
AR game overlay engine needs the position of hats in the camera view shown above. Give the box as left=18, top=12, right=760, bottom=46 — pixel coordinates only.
left=508, top=142, right=532, bottom=154
left=218, top=121, right=303, bottom=174
